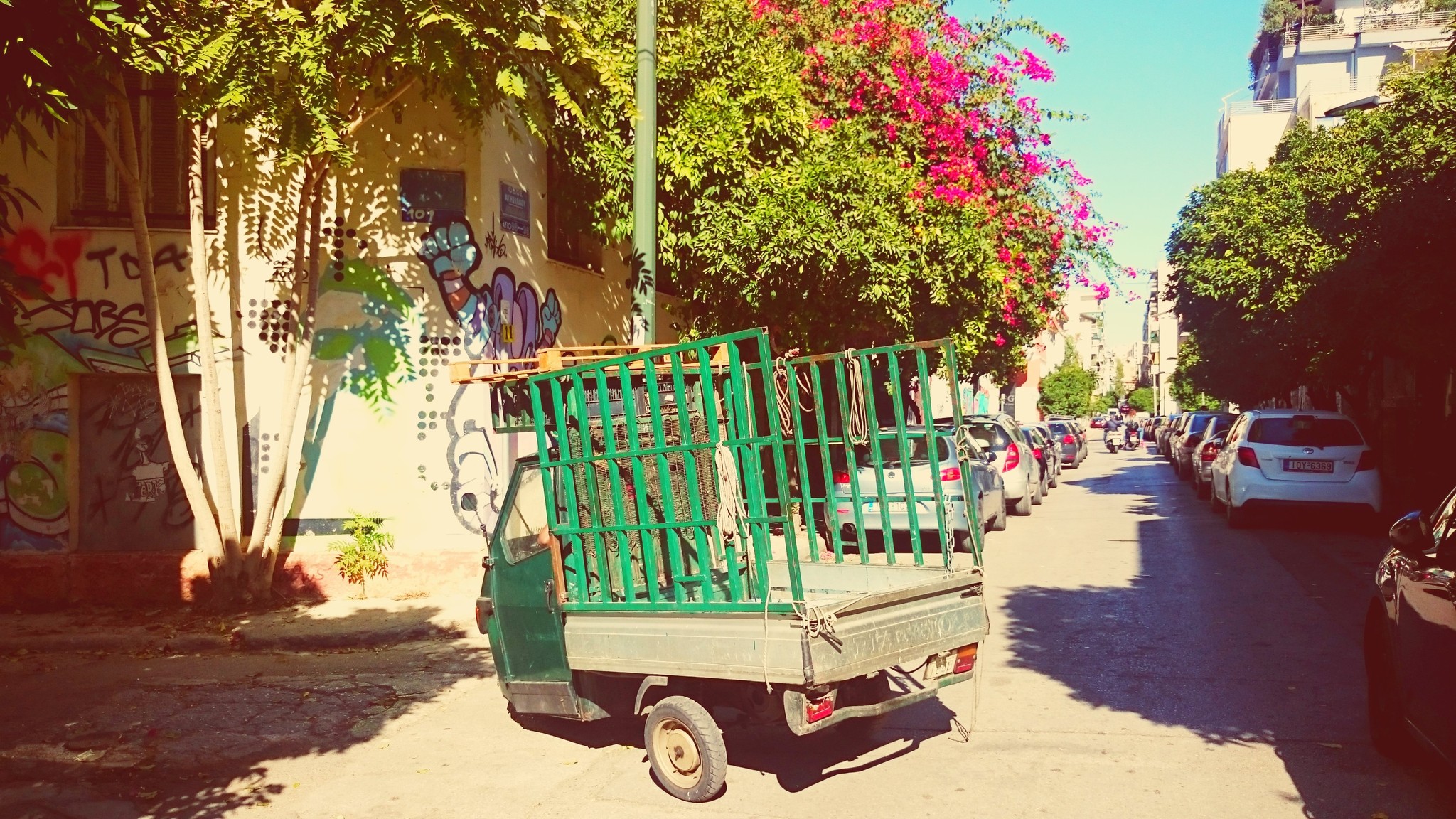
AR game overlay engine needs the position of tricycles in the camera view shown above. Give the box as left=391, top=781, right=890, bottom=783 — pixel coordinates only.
left=461, top=326, right=990, bottom=802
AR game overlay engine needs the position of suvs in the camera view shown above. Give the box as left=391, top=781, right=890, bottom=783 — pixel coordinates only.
left=929, top=414, right=1042, bottom=516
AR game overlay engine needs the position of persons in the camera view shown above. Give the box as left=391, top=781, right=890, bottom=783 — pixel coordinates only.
left=1103, top=414, right=1121, bottom=441
left=1122, top=408, right=1144, bottom=450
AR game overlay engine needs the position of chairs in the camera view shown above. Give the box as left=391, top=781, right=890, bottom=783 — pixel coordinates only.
left=913, top=440, right=943, bottom=461
left=969, top=427, right=992, bottom=447
left=1053, top=426, right=1062, bottom=432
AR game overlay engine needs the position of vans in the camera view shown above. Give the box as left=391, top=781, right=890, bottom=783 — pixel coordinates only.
left=1107, top=408, right=1121, bottom=420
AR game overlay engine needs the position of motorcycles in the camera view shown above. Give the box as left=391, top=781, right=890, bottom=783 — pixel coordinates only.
left=1102, top=422, right=1140, bottom=453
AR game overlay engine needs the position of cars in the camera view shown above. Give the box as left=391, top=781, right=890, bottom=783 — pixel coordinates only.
left=1120, top=404, right=1130, bottom=415
left=1143, top=414, right=1182, bottom=461
left=1191, top=414, right=1242, bottom=497
left=1018, top=414, right=1088, bottom=496
left=1211, top=409, right=1386, bottom=532
left=1164, top=411, right=1220, bottom=480
left=1089, top=414, right=1111, bottom=428
left=824, top=424, right=1006, bottom=553
left=1364, top=487, right=1456, bottom=773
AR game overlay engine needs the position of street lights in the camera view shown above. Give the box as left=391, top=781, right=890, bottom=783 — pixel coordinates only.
left=1127, top=355, right=1157, bottom=417
